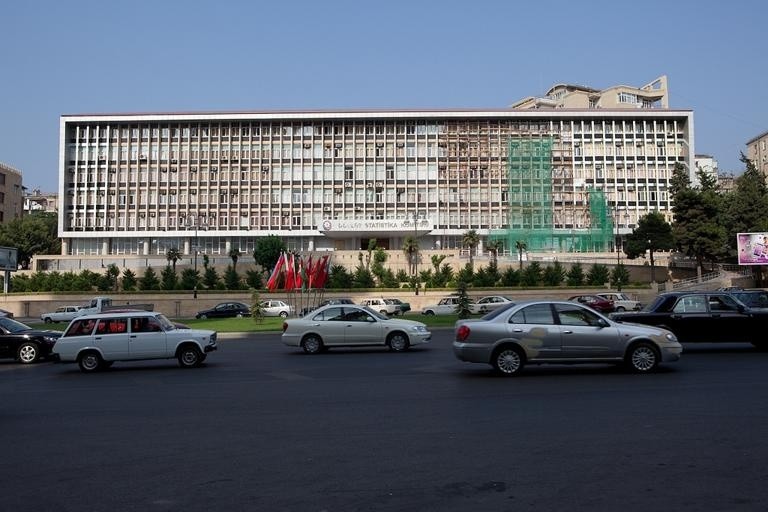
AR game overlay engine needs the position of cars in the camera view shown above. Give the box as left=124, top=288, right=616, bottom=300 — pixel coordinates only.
left=0, top=308, right=13, bottom=319
left=298, top=298, right=355, bottom=316
left=51, top=311, right=218, bottom=374
left=86, top=308, right=190, bottom=333
left=40, top=305, right=82, bottom=324
left=0, top=317, right=63, bottom=364
left=194, top=301, right=251, bottom=320
left=359, top=299, right=400, bottom=316
left=452, top=300, right=684, bottom=376
left=421, top=296, right=512, bottom=317
left=595, top=292, right=643, bottom=313
left=566, top=295, right=616, bottom=314
left=281, top=303, right=432, bottom=354
left=607, top=290, right=767, bottom=350
left=251, top=299, right=296, bottom=318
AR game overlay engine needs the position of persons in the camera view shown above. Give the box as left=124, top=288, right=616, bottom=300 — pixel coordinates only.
left=142, top=317, right=153, bottom=330
left=761, top=237, right=768, bottom=257
left=578, top=298, right=581, bottom=302
left=739, top=235, right=752, bottom=258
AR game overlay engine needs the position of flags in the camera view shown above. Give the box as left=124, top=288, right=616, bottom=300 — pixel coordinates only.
left=265, top=254, right=331, bottom=292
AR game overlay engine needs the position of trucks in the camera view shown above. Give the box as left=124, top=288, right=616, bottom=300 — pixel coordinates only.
left=72, top=297, right=154, bottom=320
